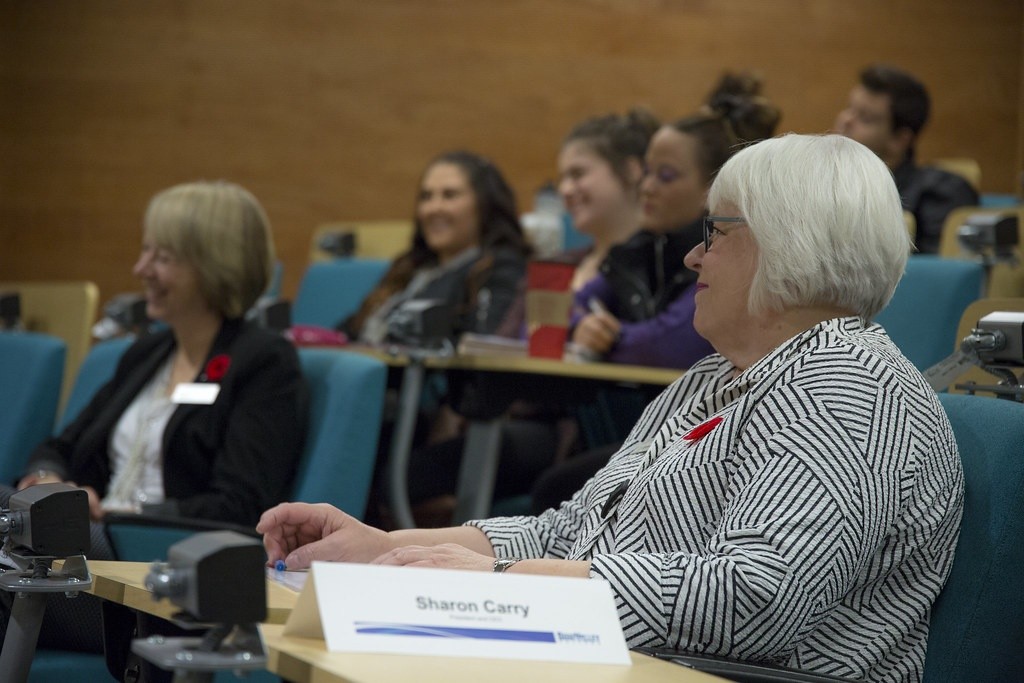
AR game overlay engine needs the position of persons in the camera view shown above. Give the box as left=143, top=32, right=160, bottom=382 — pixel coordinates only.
left=556, top=108, right=663, bottom=302
left=523, top=71, right=782, bottom=521
left=258, top=135, right=965, bottom=683
left=328, top=149, right=534, bottom=523
left=15, top=180, right=314, bottom=536
left=830, top=66, right=982, bottom=259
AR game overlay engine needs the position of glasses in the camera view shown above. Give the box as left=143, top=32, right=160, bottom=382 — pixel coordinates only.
left=703, top=215, right=747, bottom=253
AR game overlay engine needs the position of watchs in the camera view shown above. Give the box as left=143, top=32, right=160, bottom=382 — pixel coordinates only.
left=492, top=556, right=524, bottom=572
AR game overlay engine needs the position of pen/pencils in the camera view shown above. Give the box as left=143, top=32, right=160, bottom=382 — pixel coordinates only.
left=274, top=559, right=287, bottom=572
left=589, top=296, right=626, bottom=342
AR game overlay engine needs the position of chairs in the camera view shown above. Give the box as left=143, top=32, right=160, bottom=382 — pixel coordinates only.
left=0, top=152, right=1024, bottom=682
